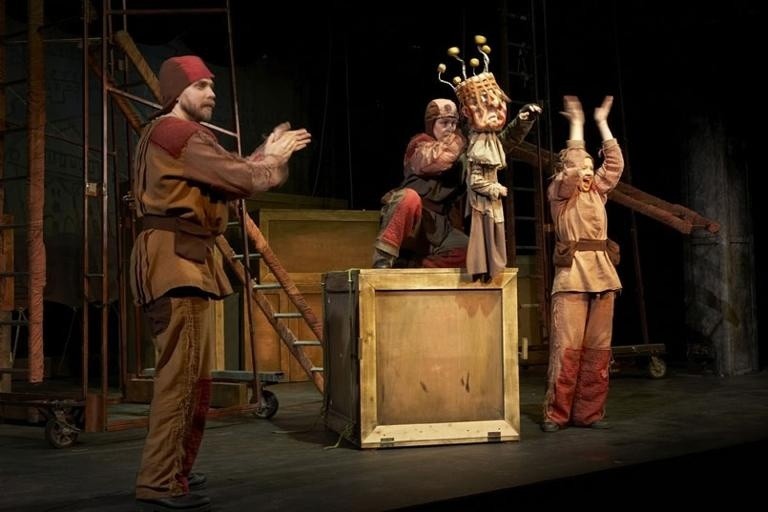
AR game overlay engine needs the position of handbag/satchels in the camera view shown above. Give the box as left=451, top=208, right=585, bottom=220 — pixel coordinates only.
left=606, top=239, right=621, bottom=267
left=174, top=222, right=211, bottom=264
left=552, top=241, right=575, bottom=268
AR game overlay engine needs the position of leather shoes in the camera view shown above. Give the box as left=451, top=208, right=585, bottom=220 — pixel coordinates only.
left=136, top=469, right=213, bottom=509
left=371, top=250, right=395, bottom=269
left=542, top=417, right=612, bottom=433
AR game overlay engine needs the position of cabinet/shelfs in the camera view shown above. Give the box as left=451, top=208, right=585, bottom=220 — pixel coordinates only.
left=318, top=262, right=520, bottom=452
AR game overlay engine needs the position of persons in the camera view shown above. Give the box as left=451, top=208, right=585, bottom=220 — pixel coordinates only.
left=541, top=95, right=625, bottom=431
left=459, top=95, right=510, bottom=283
left=369, top=97, right=543, bottom=268
left=129, top=55, right=312, bottom=512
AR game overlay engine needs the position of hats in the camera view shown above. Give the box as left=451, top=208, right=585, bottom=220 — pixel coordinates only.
left=147, top=55, right=214, bottom=120
left=423, top=97, right=460, bottom=122
